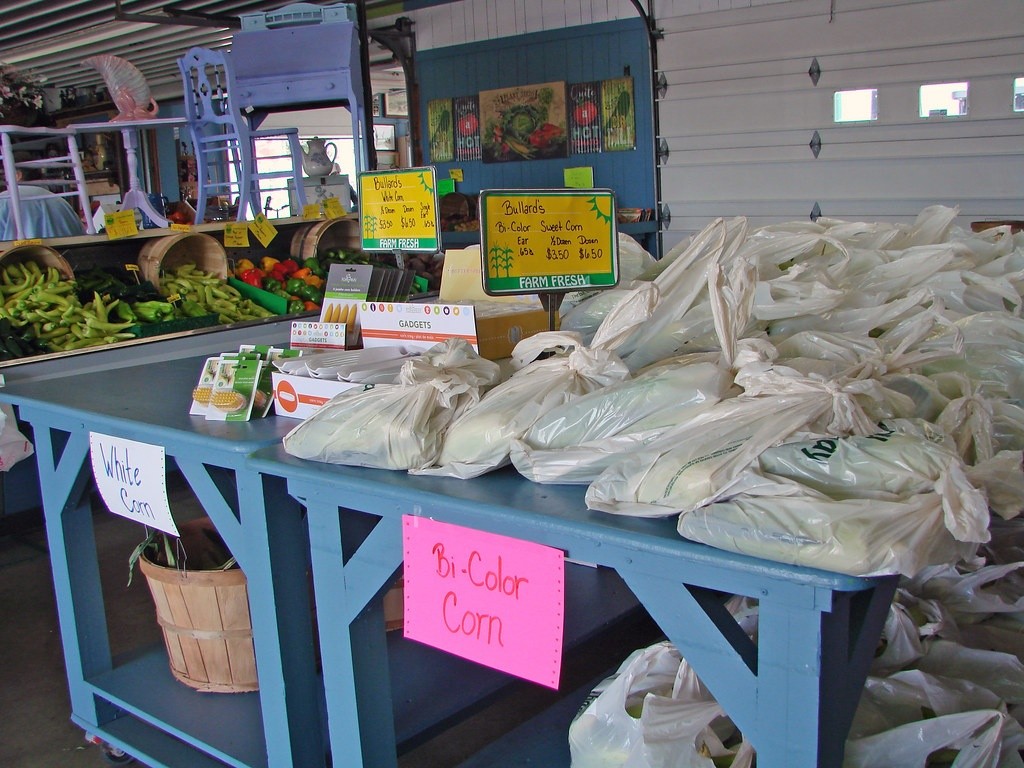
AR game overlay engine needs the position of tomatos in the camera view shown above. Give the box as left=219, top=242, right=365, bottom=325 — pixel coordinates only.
left=458, top=109, right=478, bottom=136
left=574, top=98, right=597, bottom=125
left=530, top=123, right=561, bottom=148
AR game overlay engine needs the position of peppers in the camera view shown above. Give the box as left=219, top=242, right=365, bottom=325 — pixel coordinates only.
left=0, top=247, right=394, bottom=362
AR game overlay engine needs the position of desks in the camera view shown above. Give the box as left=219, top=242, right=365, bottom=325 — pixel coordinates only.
left=0, top=342, right=904, bottom=768
left=70, top=117, right=189, bottom=226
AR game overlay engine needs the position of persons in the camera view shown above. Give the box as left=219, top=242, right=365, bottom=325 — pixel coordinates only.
left=1, top=151, right=86, bottom=239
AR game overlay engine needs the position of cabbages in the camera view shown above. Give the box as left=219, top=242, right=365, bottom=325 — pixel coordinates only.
left=501, top=104, right=536, bottom=136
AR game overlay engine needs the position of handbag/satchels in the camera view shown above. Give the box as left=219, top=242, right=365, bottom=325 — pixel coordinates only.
left=282, top=339, right=497, bottom=470
left=558, top=202, right=1024, bottom=768
left=584, top=360, right=916, bottom=518
left=509, top=255, right=779, bottom=484
left=408, top=332, right=629, bottom=480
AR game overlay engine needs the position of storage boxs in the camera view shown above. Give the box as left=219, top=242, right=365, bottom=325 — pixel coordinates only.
left=92, top=203, right=143, bottom=233
left=359, top=300, right=561, bottom=360
left=270, top=370, right=391, bottom=422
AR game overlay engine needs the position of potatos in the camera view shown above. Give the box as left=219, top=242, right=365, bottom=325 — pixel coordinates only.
left=409, top=251, right=443, bottom=291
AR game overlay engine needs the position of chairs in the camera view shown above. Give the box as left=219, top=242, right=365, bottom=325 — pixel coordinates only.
left=177, top=45, right=308, bottom=225
left=0, top=124, right=97, bottom=242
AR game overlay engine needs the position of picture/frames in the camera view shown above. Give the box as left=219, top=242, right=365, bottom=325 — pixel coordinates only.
left=373, top=124, right=397, bottom=151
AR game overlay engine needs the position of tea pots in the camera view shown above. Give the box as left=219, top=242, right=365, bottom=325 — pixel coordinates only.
left=300, top=136, right=337, bottom=177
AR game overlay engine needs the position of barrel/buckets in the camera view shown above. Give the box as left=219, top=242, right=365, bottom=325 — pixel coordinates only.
left=138, top=518, right=260, bottom=692
left=138, top=233, right=229, bottom=288
left=0, top=244, right=75, bottom=285
left=291, top=219, right=371, bottom=262
